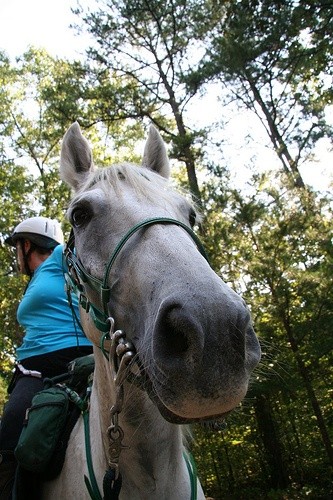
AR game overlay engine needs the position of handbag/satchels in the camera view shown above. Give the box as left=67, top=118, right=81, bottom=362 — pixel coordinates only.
left=14, top=352, right=95, bottom=481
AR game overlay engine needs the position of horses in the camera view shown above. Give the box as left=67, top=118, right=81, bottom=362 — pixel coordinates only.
left=45, top=123, right=261, bottom=500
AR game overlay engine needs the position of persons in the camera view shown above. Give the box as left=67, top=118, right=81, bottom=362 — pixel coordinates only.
left=0, top=217, right=94, bottom=500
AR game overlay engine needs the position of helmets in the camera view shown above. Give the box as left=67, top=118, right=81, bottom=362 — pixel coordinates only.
left=4, top=217, right=64, bottom=250
